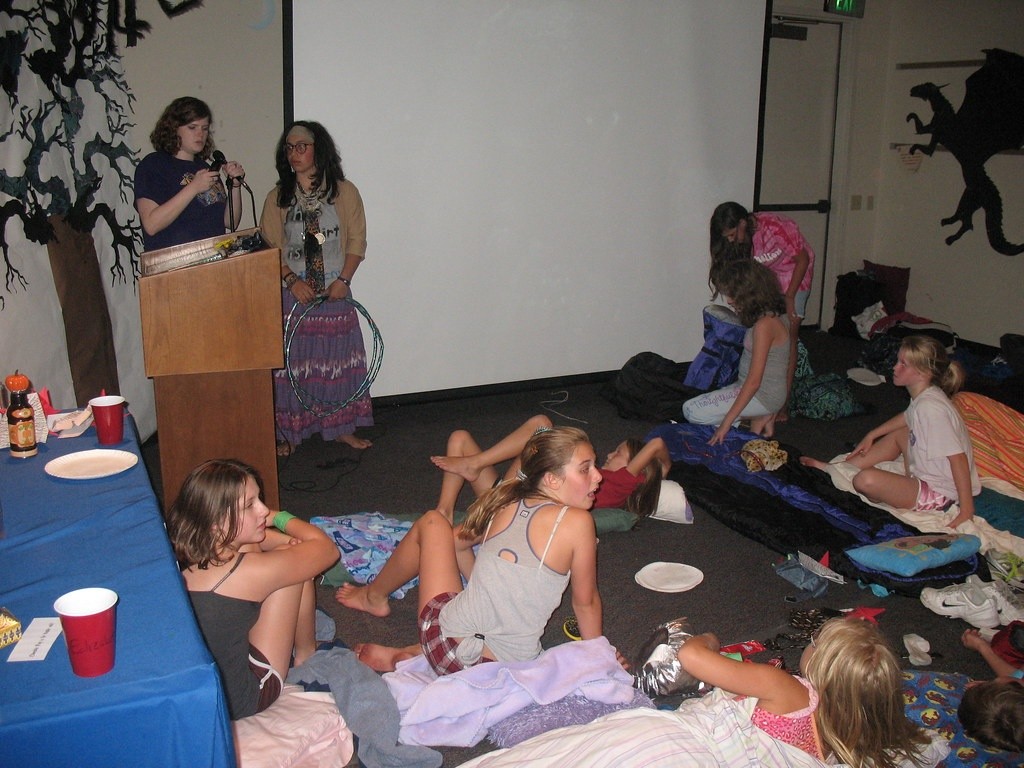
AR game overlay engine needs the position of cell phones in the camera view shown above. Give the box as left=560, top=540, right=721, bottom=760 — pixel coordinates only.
left=209, top=162, right=222, bottom=172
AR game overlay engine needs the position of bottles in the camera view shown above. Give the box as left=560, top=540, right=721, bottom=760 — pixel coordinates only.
left=5, top=374, right=38, bottom=458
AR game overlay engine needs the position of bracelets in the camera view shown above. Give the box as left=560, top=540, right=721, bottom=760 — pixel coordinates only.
left=273, top=510, right=297, bottom=533
left=284, top=272, right=299, bottom=289
left=337, top=277, right=350, bottom=286
left=233, top=185, right=241, bottom=188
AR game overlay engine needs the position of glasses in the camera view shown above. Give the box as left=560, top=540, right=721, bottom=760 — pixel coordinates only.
left=284, top=142, right=314, bottom=155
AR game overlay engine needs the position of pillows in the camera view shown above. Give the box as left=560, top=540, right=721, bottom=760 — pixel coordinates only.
left=846, top=533, right=980, bottom=577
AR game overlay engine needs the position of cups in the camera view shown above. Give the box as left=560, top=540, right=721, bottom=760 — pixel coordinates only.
left=54, top=587, right=119, bottom=677
left=88, top=395, right=126, bottom=445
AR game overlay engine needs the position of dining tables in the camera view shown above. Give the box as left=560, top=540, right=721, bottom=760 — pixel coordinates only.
left=0, top=404, right=237, bottom=768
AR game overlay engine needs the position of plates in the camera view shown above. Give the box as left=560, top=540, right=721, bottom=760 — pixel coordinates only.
left=44, top=448, right=139, bottom=480
left=634, top=562, right=704, bottom=593
left=847, top=368, right=882, bottom=386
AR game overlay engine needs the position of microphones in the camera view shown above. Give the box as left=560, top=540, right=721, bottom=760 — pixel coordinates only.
left=213, top=150, right=252, bottom=195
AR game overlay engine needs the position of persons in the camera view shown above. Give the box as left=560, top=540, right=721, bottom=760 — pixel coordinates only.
left=683, top=258, right=791, bottom=447
left=709, top=201, right=815, bottom=422
left=164, top=459, right=341, bottom=720
left=430, top=414, right=671, bottom=527
left=799, top=335, right=981, bottom=529
left=678, top=615, right=931, bottom=768
left=259, top=121, right=374, bottom=455
left=132, top=97, right=245, bottom=252
left=957, top=628, right=1024, bottom=752
left=336, top=425, right=631, bottom=679
